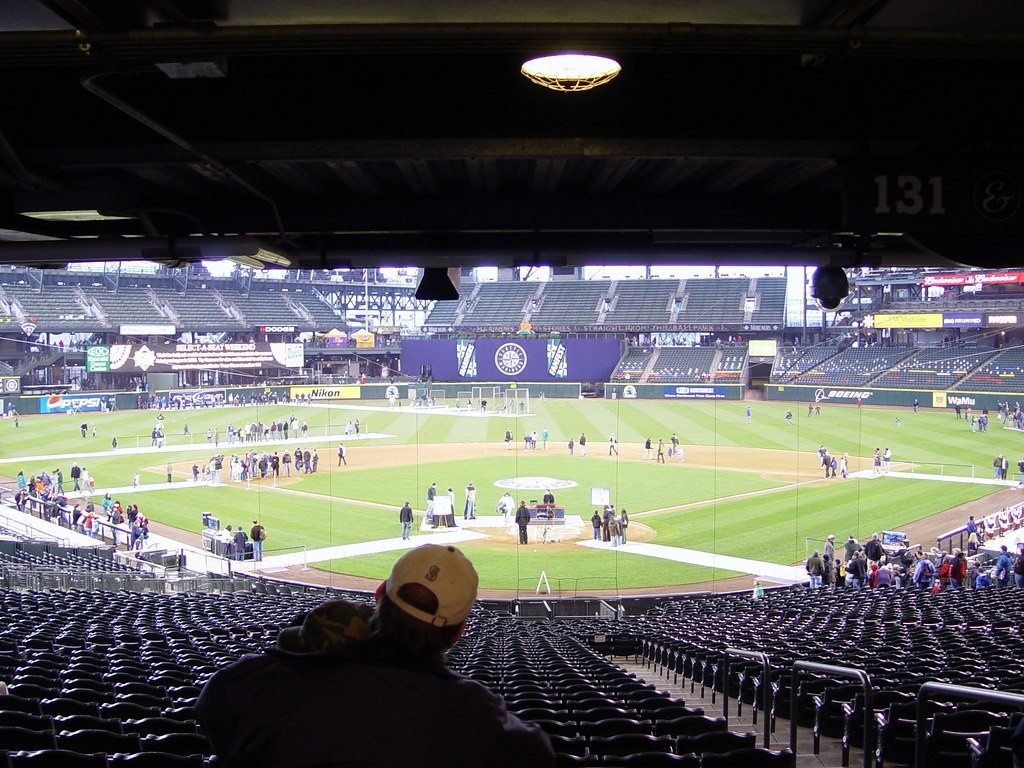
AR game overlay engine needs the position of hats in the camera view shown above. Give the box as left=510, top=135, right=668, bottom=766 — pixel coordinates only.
left=828, top=535, right=835, bottom=540
left=385, top=544, right=479, bottom=627
left=277, top=601, right=373, bottom=655
left=970, top=515, right=974, bottom=519
left=999, top=545, right=1007, bottom=551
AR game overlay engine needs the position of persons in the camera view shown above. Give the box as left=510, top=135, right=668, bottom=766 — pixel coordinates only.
left=6, top=334, right=1024, bottom=599
left=193, top=544, right=552, bottom=768
left=275, top=599, right=377, bottom=659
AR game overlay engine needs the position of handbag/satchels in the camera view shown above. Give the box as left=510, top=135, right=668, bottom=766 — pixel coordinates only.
left=259, top=529, right=265, bottom=540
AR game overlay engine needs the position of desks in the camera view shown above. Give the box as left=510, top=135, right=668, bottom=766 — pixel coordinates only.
left=528, top=506, right=565, bottom=521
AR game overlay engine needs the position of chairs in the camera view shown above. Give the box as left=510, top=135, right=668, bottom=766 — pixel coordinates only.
left=0, top=278, right=1024, bottom=768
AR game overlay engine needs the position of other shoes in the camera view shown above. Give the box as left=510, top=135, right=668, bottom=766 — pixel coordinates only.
left=464, top=517, right=467, bottom=519
left=403, top=537, right=405, bottom=540
left=469, top=517, right=475, bottom=519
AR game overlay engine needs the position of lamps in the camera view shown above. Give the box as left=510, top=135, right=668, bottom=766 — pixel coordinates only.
left=520, top=54, right=622, bottom=93
left=811, top=267, right=850, bottom=310
left=415, top=267, right=460, bottom=301
